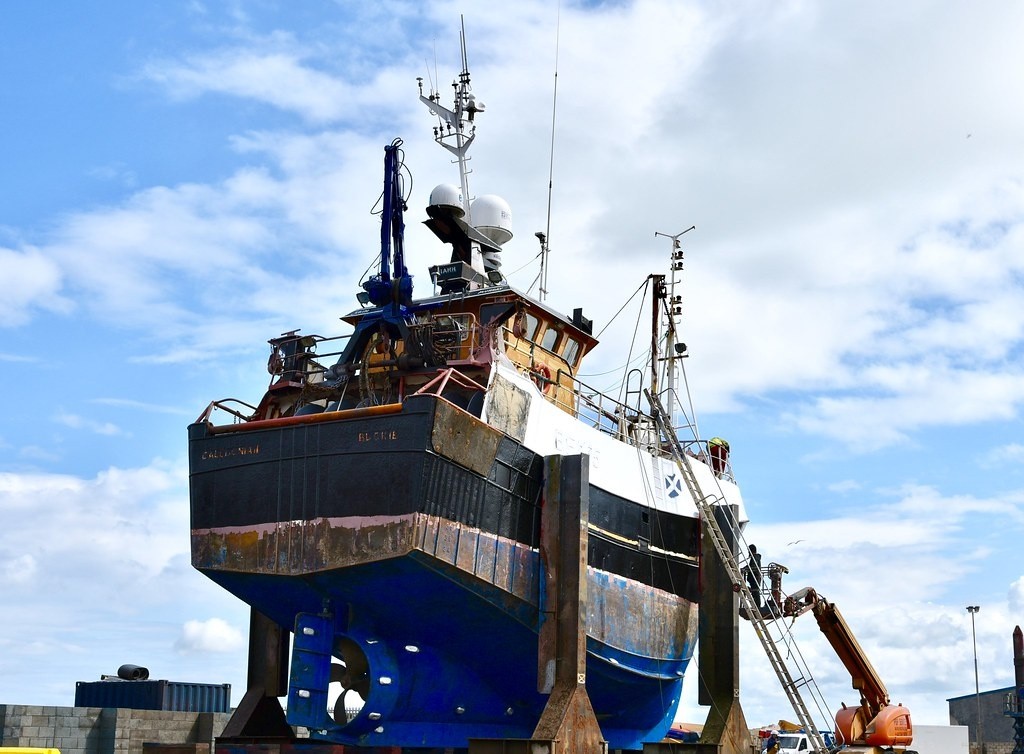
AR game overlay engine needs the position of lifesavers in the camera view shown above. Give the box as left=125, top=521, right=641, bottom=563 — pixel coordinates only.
left=532, top=363, right=553, bottom=394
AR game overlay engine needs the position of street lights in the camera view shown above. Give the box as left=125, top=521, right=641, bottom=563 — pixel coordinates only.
left=966, top=605, right=983, bottom=754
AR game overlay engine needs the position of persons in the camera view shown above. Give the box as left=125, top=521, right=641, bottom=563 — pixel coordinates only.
left=741, top=543, right=761, bottom=609
left=766, top=731, right=781, bottom=754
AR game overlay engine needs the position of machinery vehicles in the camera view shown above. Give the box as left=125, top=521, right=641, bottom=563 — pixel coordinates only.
left=738, top=562, right=918, bottom=754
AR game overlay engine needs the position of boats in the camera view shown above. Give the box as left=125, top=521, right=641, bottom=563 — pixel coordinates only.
left=187, top=14, right=751, bottom=753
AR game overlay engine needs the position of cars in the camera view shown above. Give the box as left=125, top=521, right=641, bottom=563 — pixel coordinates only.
left=762, top=734, right=825, bottom=754
